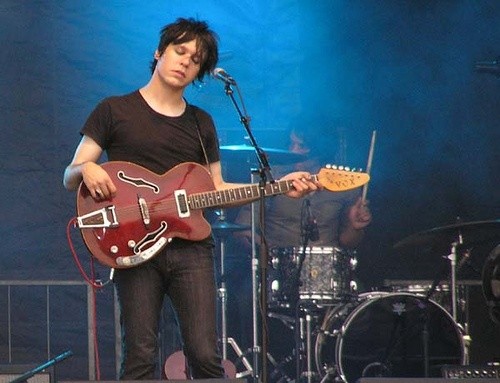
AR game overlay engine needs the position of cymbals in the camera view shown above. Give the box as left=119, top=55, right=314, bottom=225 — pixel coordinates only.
left=219, top=145, right=306, bottom=164
left=202, top=219, right=252, bottom=236
left=392, top=220, right=499, bottom=256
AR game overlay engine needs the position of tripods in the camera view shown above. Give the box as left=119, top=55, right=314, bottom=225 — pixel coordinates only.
left=215, top=157, right=321, bottom=383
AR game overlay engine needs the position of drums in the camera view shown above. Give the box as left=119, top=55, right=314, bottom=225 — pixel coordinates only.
left=390, top=283, right=466, bottom=319
left=263, top=244, right=360, bottom=319
left=314, top=290, right=467, bottom=382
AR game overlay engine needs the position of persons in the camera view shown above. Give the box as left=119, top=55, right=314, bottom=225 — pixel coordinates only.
left=63, top=18, right=323, bottom=382
left=233, top=113, right=373, bottom=383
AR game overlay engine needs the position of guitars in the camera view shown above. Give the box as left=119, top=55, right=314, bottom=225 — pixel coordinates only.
left=74, top=158, right=372, bottom=272
left=165, top=351, right=238, bottom=382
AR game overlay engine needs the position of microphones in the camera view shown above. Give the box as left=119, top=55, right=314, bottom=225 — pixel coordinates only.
left=213, top=67, right=236, bottom=86
left=456, top=246, right=473, bottom=271
left=306, top=199, right=320, bottom=241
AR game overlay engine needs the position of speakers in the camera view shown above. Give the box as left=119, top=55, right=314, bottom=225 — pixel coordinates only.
left=0, top=364, right=56, bottom=383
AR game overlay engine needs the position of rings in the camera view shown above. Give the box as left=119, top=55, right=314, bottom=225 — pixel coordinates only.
left=95, top=189, right=103, bottom=197
